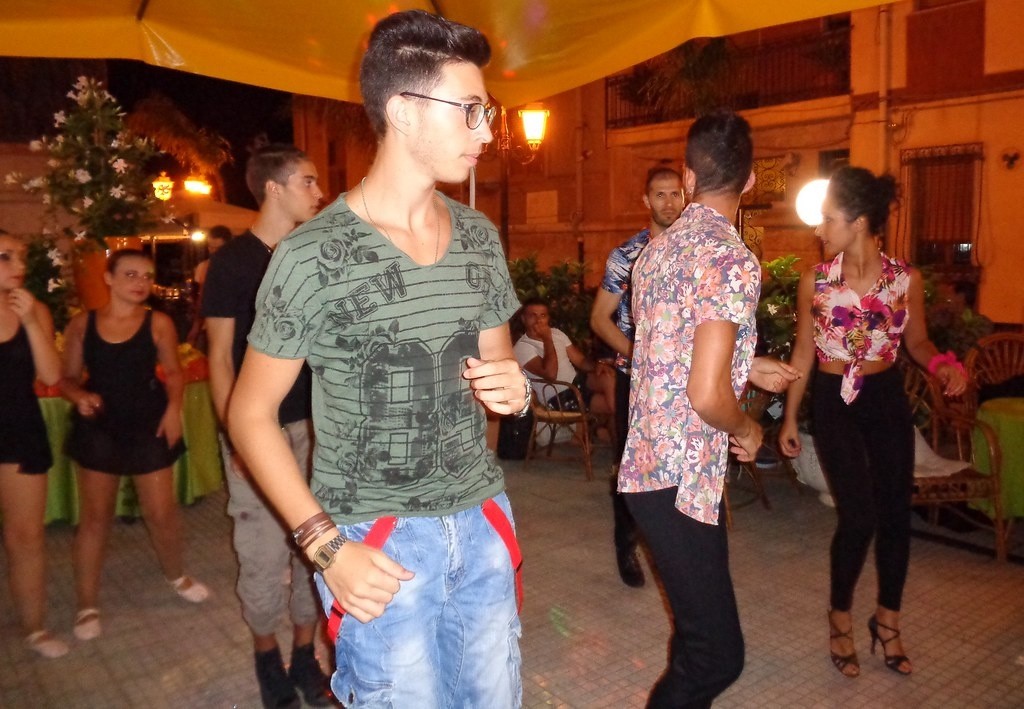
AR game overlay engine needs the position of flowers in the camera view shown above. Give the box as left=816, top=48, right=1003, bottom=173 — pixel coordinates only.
left=4, top=75, right=192, bottom=287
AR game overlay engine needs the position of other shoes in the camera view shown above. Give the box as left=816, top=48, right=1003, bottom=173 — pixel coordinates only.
left=73, top=607, right=103, bottom=640
left=21, top=628, right=71, bottom=660
left=163, top=571, right=208, bottom=603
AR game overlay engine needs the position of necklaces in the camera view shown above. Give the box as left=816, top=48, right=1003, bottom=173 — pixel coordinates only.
left=360, top=177, right=440, bottom=266
left=251, top=226, right=273, bottom=255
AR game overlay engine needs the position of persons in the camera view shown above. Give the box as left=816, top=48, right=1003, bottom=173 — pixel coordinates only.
left=591, top=113, right=804, bottom=709
left=780, top=165, right=968, bottom=679
left=0, top=230, right=71, bottom=656
left=53, top=248, right=210, bottom=640
left=512, top=301, right=617, bottom=453
left=194, top=9, right=531, bottom=709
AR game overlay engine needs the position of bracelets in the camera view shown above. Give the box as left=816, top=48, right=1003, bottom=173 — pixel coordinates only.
left=928, top=350, right=969, bottom=378
left=291, top=512, right=335, bottom=554
left=514, top=369, right=533, bottom=417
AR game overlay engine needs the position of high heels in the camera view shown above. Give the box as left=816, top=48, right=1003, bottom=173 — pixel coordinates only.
left=825, top=609, right=912, bottom=679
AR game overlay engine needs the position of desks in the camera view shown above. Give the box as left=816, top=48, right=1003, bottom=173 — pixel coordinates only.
left=967, top=398, right=1024, bottom=521
left=33, top=340, right=220, bottom=530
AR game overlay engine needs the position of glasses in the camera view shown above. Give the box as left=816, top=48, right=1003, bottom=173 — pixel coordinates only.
left=399, top=91, right=497, bottom=130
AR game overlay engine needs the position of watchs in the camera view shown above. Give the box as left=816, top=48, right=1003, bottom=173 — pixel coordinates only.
left=314, top=534, right=347, bottom=575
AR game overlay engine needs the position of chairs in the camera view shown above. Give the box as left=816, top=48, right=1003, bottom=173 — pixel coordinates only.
left=960, top=331, right=1024, bottom=419
left=721, top=382, right=771, bottom=530
left=522, top=378, right=595, bottom=480
left=898, top=355, right=1005, bottom=563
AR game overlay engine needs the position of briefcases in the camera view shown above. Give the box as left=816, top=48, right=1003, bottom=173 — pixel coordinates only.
left=497, top=406, right=534, bottom=462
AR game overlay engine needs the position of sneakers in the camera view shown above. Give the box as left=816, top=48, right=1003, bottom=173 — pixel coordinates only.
left=254, top=642, right=335, bottom=709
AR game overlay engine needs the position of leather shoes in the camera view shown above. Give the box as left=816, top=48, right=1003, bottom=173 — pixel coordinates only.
left=616, top=546, right=646, bottom=588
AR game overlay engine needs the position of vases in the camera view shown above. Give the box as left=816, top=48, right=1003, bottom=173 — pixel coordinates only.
left=80, top=236, right=144, bottom=309
